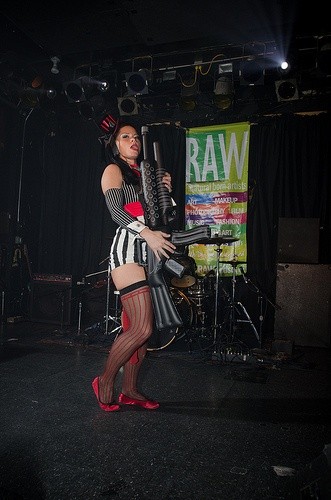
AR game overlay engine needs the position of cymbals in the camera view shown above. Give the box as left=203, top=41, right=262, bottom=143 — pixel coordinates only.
left=196, top=233, right=239, bottom=244
left=218, top=260, right=250, bottom=264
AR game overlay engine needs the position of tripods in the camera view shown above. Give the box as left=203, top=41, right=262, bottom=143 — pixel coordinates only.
left=188, top=245, right=267, bottom=366
left=80, top=268, right=124, bottom=336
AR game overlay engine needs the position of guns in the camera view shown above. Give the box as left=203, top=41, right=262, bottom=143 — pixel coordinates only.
left=136, top=126, right=211, bottom=332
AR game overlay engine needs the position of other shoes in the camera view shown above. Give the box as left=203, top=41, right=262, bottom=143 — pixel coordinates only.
left=92, top=376, right=120, bottom=412
left=117, top=393, right=160, bottom=409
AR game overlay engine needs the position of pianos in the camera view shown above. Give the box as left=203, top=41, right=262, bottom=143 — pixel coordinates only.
left=20, top=271, right=78, bottom=330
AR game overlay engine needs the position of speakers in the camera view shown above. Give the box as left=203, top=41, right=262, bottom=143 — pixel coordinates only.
left=275, top=264, right=331, bottom=347
left=30, top=280, right=76, bottom=325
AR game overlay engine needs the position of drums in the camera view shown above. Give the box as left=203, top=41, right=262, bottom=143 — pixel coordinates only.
left=186, top=274, right=210, bottom=300
left=146, top=285, right=193, bottom=352
left=168, top=256, right=196, bottom=289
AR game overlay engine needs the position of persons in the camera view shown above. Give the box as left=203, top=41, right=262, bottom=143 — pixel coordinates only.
left=92, top=121, right=177, bottom=411
